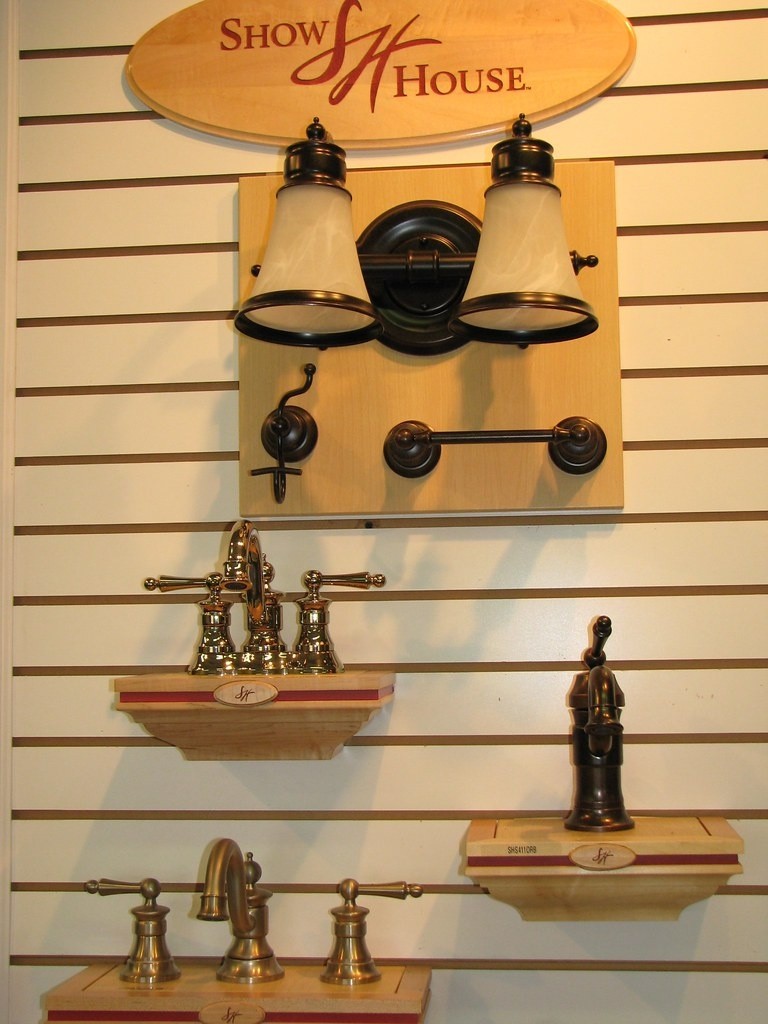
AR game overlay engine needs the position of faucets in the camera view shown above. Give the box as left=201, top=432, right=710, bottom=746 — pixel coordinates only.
left=221, top=519, right=288, bottom=675
left=563, top=616, right=635, bottom=833
left=196, top=838, right=286, bottom=984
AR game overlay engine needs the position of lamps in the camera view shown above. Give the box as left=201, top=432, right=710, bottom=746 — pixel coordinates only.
left=446, top=113, right=600, bottom=350
left=235, top=118, right=381, bottom=348
